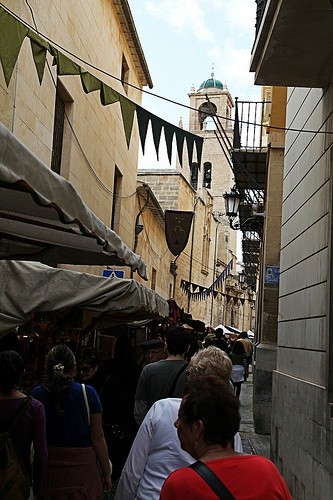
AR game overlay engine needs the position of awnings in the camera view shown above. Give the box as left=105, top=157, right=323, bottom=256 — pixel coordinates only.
left=0, top=259, right=168, bottom=332
left=0, top=124, right=147, bottom=278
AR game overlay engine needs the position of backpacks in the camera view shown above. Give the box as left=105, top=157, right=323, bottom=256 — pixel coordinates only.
left=0, top=396, right=32, bottom=500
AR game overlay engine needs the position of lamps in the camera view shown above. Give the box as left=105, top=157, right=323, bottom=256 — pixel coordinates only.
left=214, top=259, right=221, bottom=271
left=239, top=270, right=256, bottom=290
left=223, top=187, right=264, bottom=230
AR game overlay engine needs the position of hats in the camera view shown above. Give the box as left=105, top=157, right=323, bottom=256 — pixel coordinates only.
left=140, top=340, right=165, bottom=349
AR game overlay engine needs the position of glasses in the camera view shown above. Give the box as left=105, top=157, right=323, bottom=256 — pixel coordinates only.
left=185, top=369, right=191, bottom=377
left=75, top=366, right=92, bottom=374
left=174, top=420, right=182, bottom=428
left=143, top=350, right=162, bottom=356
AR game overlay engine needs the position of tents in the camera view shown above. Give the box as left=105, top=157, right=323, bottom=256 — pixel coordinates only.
left=213, top=324, right=255, bottom=338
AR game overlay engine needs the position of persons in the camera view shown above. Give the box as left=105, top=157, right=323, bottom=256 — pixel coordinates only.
left=0, top=327, right=293, bottom=500
